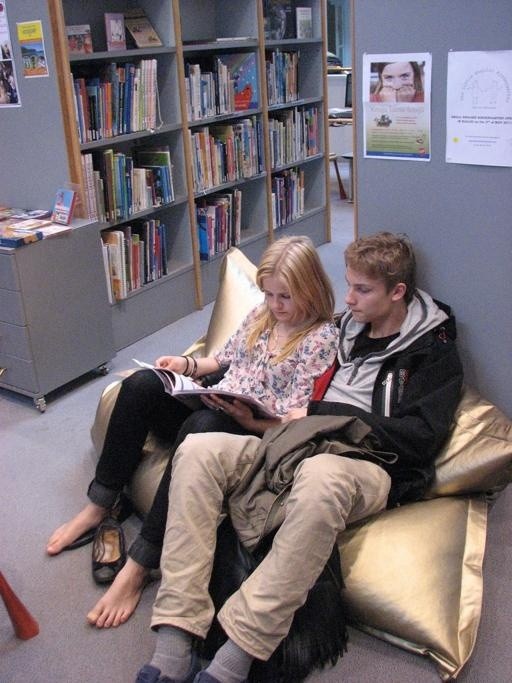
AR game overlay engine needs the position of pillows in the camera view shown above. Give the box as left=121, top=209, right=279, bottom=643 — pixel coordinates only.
left=90, top=248, right=512, bottom=680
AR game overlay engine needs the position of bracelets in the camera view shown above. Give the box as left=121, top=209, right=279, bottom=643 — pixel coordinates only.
left=180, top=354, right=189, bottom=377
left=189, top=353, right=197, bottom=379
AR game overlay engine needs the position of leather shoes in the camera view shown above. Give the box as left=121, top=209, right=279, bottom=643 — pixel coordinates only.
left=93, top=517, right=128, bottom=586
left=62, top=493, right=132, bottom=550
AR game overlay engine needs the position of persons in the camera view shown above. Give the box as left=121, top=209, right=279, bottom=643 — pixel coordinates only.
left=49, top=231, right=338, bottom=628
left=130, top=229, right=461, bottom=682
left=371, top=61, right=425, bottom=103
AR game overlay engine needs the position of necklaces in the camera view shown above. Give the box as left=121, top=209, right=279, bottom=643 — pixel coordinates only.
left=271, top=320, right=299, bottom=359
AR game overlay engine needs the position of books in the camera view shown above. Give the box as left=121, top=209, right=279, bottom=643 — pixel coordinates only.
left=188, top=116, right=264, bottom=192
left=81, top=145, right=176, bottom=223
left=65, top=7, right=163, bottom=53
left=272, top=166, right=305, bottom=229
left=128, top=350, right=287, bottom=422
left=269, top=103, right=320, bottom=168
left=1, top=187, right=77, bottom=247
left=70, top=59, right=158, bottom=143
left=195, top=189, right=242, bottom=262
left=265, top=47, right=301, bottom=105
left=184, top=51, right=260, bottom=122
left=263, top=2, right=312, bottom=39
left=101, top=219, right=168, bottom=304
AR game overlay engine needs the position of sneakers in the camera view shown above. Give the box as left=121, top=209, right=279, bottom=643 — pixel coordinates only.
left=194, top=667, right=253, bottom=682
left=132, top=649, right=200, bottom=683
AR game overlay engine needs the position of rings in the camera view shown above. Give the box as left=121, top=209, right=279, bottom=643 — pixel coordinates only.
left=215, top=406, right=225, bottom=411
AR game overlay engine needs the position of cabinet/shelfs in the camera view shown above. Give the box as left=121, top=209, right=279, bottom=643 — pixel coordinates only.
left=0, top=1, right=331, bottom=352
left=1, top=217, right=117, bottom=412
